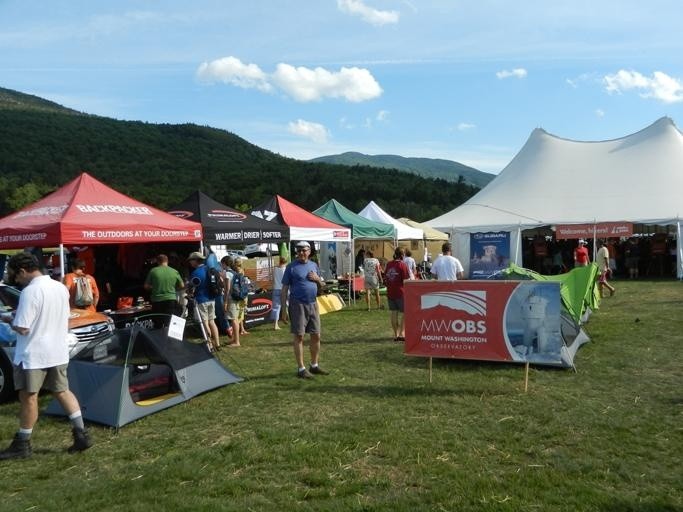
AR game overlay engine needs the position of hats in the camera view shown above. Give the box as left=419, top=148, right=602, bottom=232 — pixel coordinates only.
left=296, top=241, right=311, bottom=247
left=190, top=252, right=206, bottom=259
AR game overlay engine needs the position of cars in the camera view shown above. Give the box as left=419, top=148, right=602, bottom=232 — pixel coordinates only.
left=0, top=283, right=116, bottom=404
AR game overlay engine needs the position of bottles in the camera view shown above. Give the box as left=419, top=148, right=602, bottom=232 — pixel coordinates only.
left=137, top=296, right=144, bottom=309
left=210, top=275, right=215, bottom=282
left=234, top=278, right=239, bottom=288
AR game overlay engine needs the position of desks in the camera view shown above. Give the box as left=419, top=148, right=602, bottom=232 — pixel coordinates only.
left=240, top=288, right=283, bottom=330
left=336, top=275, right=365, bottom=301
left=107, top=305, right=154, bottom=330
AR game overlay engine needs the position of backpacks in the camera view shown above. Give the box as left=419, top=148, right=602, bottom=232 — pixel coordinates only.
left=226, top=269, right=249, bottom=301
left=206, top=267, right=224, bottom=298
left=72, top=271, right=94, bottom=307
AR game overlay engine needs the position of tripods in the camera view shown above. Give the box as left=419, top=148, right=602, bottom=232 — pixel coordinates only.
left=179, top=296, right=212, bottom=352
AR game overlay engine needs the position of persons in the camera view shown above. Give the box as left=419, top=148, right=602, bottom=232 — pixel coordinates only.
left=522, top=233, right=677, bottom=298
left=143, top=254, right=185, bottom=329
left=49, top=245, right=124, bottom=313
left=269, top=255, right=290, bottom=330
left=384, top=246, right=411, bottom=341
left=363, top=250, right=383, bottom=311
left=403, top=251, right=417, bottom=280
left=280, top=241, right=329, bottom=379
left=0, top=251, right=93, bottom=459
left=186, top=251, right=256, bottom=352
left=350, top=249, right=365, bottom=300
left=429, top=243, right=464, bottom=281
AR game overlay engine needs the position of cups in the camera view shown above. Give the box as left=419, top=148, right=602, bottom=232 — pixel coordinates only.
left=244, top=276, right=247, bottom=279
left=360, top=269, right=364, bottom=277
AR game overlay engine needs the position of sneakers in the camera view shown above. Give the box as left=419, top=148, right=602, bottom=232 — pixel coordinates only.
left=67, top=431, right=94, bottom=454
left=298, top=368, right=314, bottom=379
left=310, top=366, right=330, bottom=375
left=1, top=439, right=31, bottom=459
